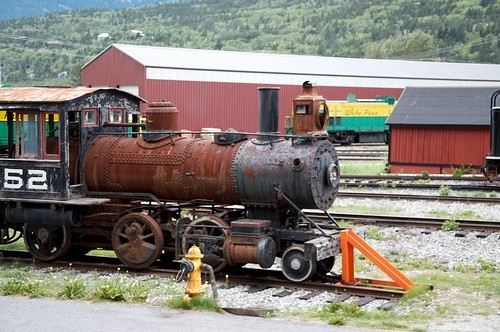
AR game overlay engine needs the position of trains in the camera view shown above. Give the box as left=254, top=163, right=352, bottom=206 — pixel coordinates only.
left=283, top=90, right=399, bottom=147
left=0, top=80, right=346, bottom=283
